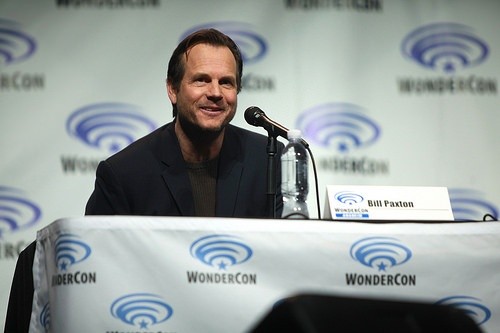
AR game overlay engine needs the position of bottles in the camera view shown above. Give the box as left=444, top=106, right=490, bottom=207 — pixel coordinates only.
left=280, top=130, right=309, bottom=219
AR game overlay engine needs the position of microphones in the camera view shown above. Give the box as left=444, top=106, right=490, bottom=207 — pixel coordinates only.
left=244, top=106, right=309, bottom=149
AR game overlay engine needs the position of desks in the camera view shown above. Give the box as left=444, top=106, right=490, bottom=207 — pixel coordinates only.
left=3, top=216, right=500, bottom=333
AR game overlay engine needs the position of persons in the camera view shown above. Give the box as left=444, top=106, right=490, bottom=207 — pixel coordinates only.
left=85, top=28, right=287, bottom=219
